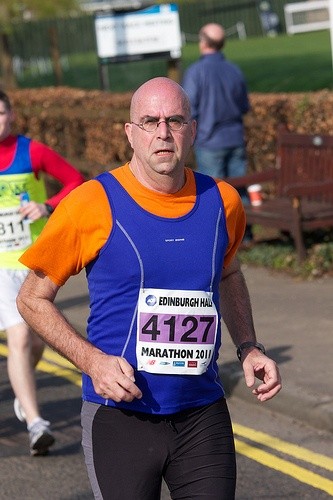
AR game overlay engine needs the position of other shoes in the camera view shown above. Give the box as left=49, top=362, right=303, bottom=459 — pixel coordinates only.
left=28, top=422, right=55, bottom=456
left=13, top=397, right=25, bottom=423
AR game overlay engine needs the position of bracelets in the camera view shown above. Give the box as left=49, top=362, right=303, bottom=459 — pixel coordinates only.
left=45, top=205, right=52, bottom=216
left=236, top=341, right=264, bottom=361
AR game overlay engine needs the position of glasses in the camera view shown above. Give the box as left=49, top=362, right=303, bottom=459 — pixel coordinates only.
left=131, top=115, right=191, bottom=132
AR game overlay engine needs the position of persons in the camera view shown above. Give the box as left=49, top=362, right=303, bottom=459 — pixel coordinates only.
left=181, top=22, right=255, bottom=247
left=0, top=88, right=84, bottom=456
left=14, top=77, right=282, bottom=500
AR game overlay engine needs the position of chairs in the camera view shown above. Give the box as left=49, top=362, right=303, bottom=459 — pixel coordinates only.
left=218, top=132, right=332, bottom=261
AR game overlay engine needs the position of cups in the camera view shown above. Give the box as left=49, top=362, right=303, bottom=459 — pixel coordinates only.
left=248, top=184, right=264, bottom=208
